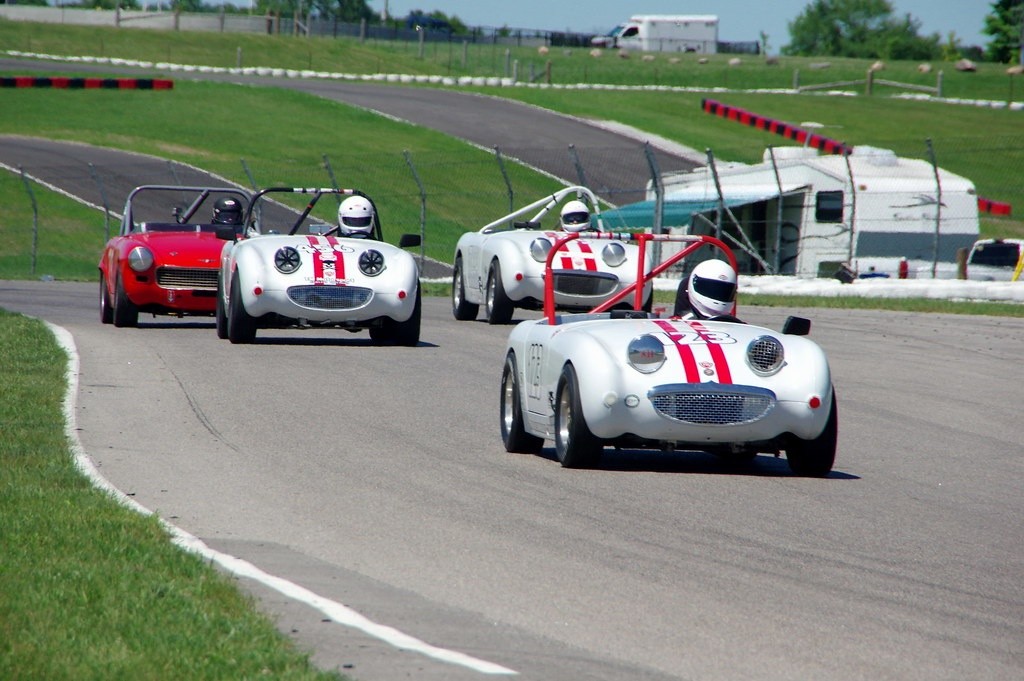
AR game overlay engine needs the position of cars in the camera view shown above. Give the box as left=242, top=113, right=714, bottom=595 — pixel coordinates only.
left=499, top=234, right=838, bottom=477
left=216, top=186, right=422, bottom=346
left=97, top=184, right=262, bottom=328
left=451, top=185, right=654, bottom=327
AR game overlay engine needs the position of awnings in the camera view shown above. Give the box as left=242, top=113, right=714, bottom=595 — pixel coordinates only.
left=587, top=197, right=773, bottom=275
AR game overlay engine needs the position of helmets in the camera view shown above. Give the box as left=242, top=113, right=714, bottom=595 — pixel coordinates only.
left=688, top=259, right=737, bottom=318
left=338, top=195, right=374, bottom=236
left=559, top=200, right=591, bottom=232
left=210, top=196, right=242, bottom=226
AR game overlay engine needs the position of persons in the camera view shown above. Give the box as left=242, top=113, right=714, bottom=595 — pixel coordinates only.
left=558, top=199, right=595, bottom=233
left=328, top=195, right=376, bottom=239
left=679, top=258, right=738, bottom=322
left=210, top=196, right=244, bottom=226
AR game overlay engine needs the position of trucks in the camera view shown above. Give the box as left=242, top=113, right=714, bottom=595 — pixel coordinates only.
left=591, top=16, right=719, bottom=55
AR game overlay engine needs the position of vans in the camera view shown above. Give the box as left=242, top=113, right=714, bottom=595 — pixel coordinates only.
left=965, top=238, right=1024, bottom=282
